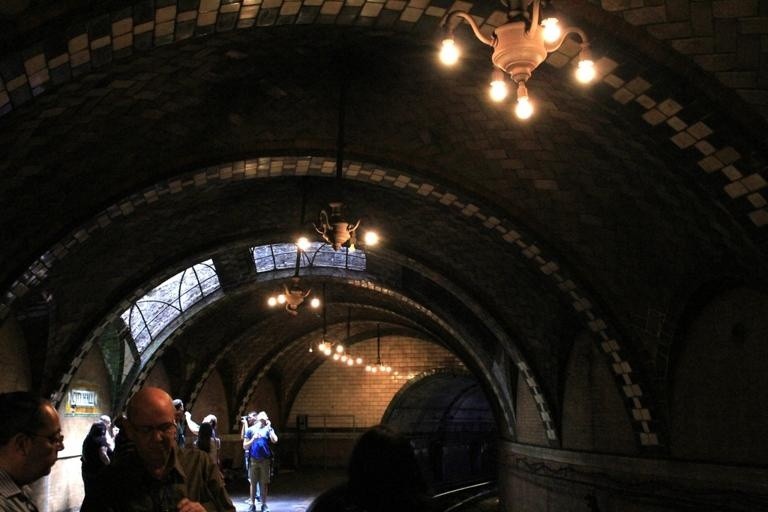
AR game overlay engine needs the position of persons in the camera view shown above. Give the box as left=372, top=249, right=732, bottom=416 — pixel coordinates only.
left=308, top=426, right=424, bottom=511
left=1, top=391, right=63, bottom=512
left=81, top=386, right=279, bottom=512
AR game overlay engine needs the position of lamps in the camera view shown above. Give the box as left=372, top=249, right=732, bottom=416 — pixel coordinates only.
left=438, top=1, right=596, bottom=122
left=264, top=49, right=383, bottom=319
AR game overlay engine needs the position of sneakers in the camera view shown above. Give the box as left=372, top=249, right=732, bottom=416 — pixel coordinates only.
left=249, top=504, right=269, bottom=512
